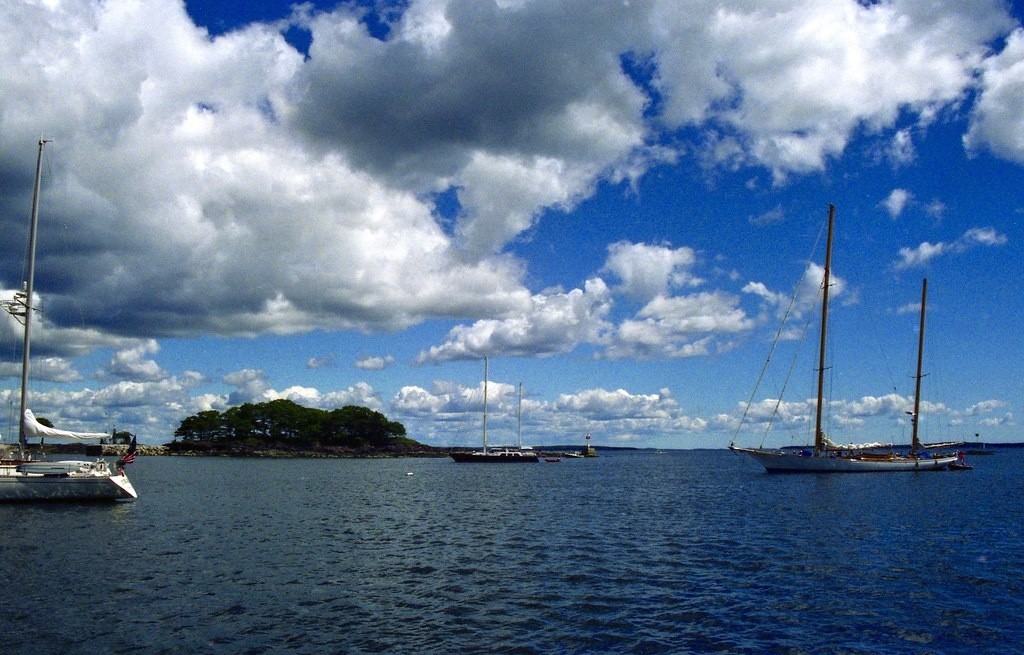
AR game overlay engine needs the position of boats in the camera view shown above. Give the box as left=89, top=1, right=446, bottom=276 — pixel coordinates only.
left=564, top=434, right=598, bottom=458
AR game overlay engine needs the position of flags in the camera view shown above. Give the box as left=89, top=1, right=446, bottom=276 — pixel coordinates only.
left=121, top=435, right=136, bottom=464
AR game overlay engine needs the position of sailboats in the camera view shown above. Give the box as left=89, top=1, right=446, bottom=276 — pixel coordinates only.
left=730, top=202, right=961, bottom=472
left=449, top=355, right=539, bottom=462
left=0, top=138, right=139, bottom=502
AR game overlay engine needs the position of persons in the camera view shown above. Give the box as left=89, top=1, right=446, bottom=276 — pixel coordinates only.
left=26, top=451, right=31, bottom=461
left=793, top=443, right=964, bottom=460
